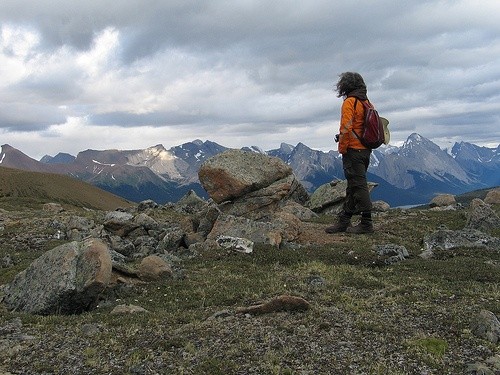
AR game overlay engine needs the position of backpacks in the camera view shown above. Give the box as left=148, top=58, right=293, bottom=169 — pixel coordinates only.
left=352, top=96, right=385, bottom=149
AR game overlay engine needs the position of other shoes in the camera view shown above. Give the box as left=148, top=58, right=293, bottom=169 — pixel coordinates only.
left=346, top=222, right=374, bottom=233
left=324, top=219, right=352, bottom=233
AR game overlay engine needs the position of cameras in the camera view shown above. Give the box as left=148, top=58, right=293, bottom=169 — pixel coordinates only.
left=335, top=134, right=339, bottom=142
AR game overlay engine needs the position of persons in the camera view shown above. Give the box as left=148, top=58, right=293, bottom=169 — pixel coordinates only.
left=325, top=73, right=373, bottom=233
